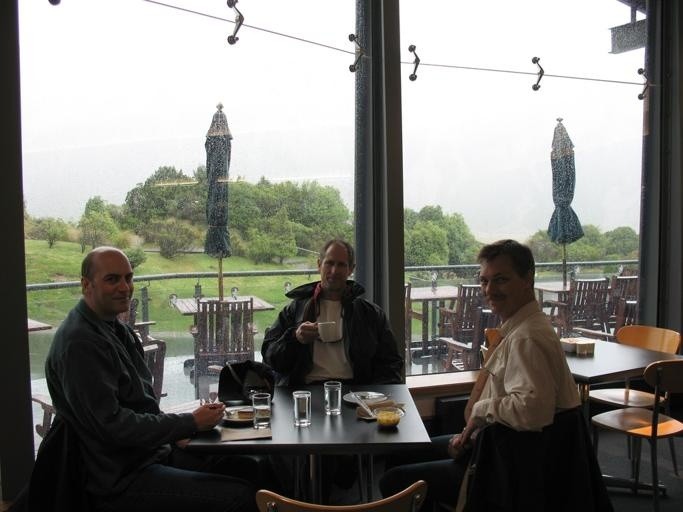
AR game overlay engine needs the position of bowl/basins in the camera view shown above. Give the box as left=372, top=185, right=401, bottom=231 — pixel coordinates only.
left=373, top=406, right=404, bottom=426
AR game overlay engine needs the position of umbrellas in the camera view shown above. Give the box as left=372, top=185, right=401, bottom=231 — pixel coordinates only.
left=205, top=103, right=232, bottom=300
left=550, top=118, right=585, bottom=289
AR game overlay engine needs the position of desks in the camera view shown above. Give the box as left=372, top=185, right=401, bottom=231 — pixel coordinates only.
left=171, top=296, right=275, bottom=379
left=27, top=317, right=53, bottom=331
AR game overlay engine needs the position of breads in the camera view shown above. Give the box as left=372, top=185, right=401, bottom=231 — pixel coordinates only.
left=238, top=406, right=270, bottom=420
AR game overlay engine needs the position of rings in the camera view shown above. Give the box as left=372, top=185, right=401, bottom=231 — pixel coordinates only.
left=463, top=427, right=465, bottom=430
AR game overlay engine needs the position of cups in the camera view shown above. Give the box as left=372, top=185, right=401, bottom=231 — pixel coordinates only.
left=313, top=321, right=336, bottom=343
left=251, top=392, right=271, bottom=429
left=322, top=380, right=342, bottom=415
left=560, top=337, right=595, bottom=354
left=291, top=390, right=312, bottom=427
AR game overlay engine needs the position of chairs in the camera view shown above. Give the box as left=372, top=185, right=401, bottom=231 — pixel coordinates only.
left=405, top=281, right=499, bottom=371
left=255, top=480, right=428, bottom=512
left=190, top=298, right=258, bottom=400
left=534, top=267, right=639, bottom=338
left=559, top=326, right=683, bottom=512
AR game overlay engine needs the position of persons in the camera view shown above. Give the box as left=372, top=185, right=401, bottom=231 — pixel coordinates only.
left=30, top=247, right=256, bottom=511
left=379, top=241, right=582, bottom=512
left=260, top=239, right=403, bottom=386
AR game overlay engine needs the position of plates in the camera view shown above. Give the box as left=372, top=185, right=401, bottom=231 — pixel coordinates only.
left=220, top=404, right=270, bottom=423
left=342, top=391, right=388, bottom=406
